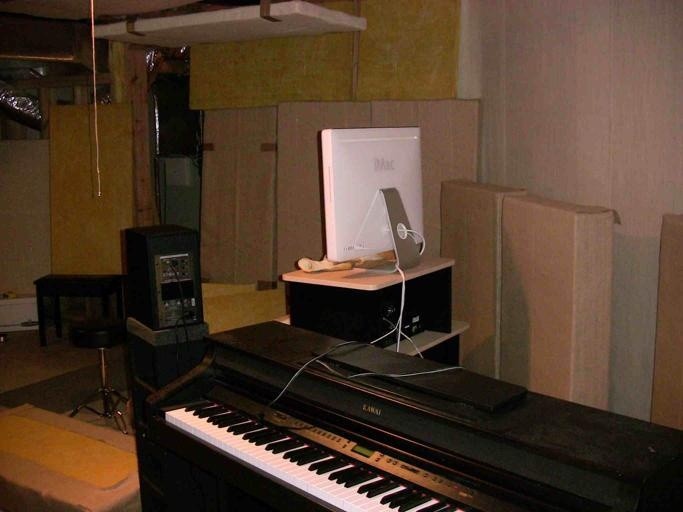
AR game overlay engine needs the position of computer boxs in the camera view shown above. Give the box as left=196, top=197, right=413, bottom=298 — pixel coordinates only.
left=121, top=223, right=205, bottom=332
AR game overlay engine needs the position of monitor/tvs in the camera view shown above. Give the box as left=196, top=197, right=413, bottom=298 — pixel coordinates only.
left=317, top=127, right=425, bottom=271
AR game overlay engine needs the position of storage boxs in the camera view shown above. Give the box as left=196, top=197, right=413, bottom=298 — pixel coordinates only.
left=500, top=192, right=618, bottom=413
left=437, top=178, right=532, bottom=380
left=650, top=210, right=682, bottom=432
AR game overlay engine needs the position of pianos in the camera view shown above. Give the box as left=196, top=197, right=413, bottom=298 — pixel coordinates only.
left=134, top=320, right=682, bottom=511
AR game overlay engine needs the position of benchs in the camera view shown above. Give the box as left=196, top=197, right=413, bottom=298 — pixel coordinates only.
left=31, top=273, right=123, bottom=350
